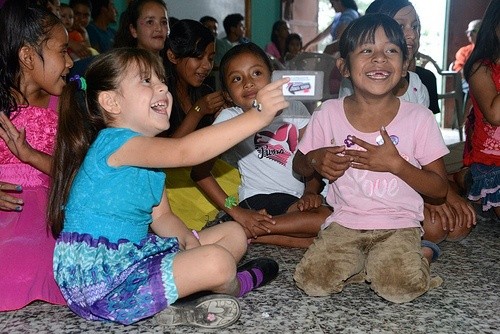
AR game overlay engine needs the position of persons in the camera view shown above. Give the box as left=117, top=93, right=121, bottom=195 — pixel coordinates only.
left=0, top=0, right=500, bottom=250
left=291, top=12, right=450, bottom=305
left=0, top=3, right=75, bottom=312
left=46, top=46, right=292, bottom=331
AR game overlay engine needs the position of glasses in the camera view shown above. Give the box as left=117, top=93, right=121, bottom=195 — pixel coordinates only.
left=466, top=30, right=477, bottom=33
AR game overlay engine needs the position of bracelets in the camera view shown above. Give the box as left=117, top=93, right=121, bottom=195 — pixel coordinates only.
left=223, top=194, right=238, bottom=212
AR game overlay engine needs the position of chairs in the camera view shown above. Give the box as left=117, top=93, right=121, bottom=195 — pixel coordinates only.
left=264, top=52, right=285, bottom=73
left=286, top=51, right=343, bottom=101
left=413, top=52, right=464, bottom=142
left=451, top=88, right=468, bottom=131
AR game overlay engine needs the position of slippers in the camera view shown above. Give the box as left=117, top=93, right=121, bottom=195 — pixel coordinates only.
left=420, top=240, right=440, bottom=259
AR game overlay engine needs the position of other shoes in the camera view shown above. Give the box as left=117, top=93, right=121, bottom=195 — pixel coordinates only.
left=154, top=294, right=240, bottom=330
left=236, top=257, right=278, bottom=287
